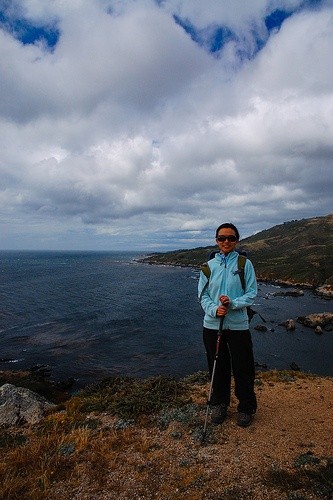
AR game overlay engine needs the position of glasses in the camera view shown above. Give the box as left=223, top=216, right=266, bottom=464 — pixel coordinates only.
left=217, top=235, right=237, bottom=242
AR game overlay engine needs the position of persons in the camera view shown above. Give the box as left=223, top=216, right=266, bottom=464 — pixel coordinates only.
left=197, top=222, right=258, bottom=427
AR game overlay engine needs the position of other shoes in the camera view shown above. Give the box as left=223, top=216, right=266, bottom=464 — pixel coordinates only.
left=238, top=411, right=255, bottom=426
left=211, top=405, right=226, bottom=423
